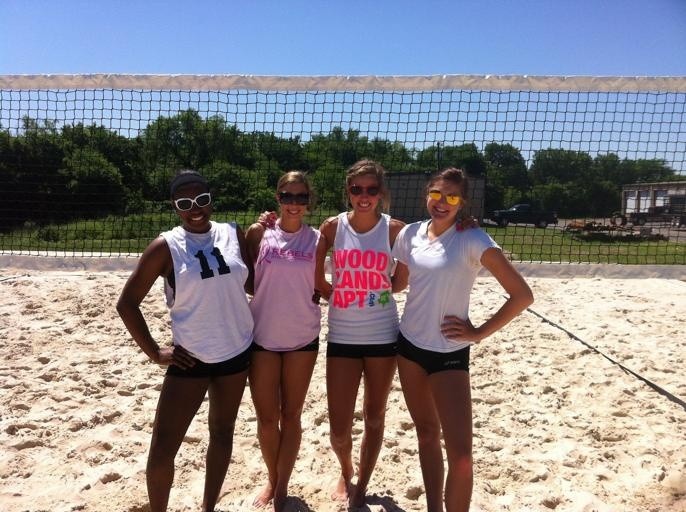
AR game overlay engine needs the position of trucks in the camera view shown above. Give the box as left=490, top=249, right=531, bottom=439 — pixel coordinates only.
left=608, top=192, right=685, bottom=230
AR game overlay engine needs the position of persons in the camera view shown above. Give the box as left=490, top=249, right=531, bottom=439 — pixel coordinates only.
left=387, top=168, right=533, bottom=512
left=243, top=172, right=334, bottom=512
left=116, top=171, right=319, bottom=512
left=318, top=158, right=407, bottom=512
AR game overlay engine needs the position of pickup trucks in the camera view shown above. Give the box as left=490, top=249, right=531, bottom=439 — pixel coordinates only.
left=490, top=204, right=559, bottom=229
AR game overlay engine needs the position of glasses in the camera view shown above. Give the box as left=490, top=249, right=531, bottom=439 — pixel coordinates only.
left=172, top=186, right=212, bottom=212
left=277, top=189, right=311, bottom=206
left=347, top=180, right=381, bottom=197
left=427, top=185, right=461, bottom=206
